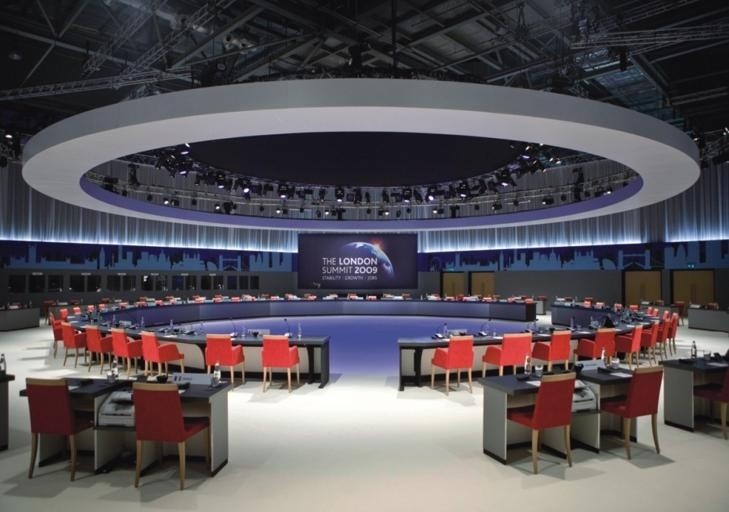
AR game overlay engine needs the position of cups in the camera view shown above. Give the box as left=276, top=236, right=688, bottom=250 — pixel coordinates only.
left=535, top=363, right=544, bottom=376
left=158, top=376, right=167, bottom=382
left=703, top=348, right=711, bottom=360
left=574, top=363, right=583, bottom=375
left=252, top=332, right=259, bottom=337
left=108, top=374, right=115, bottom=382
left=612, top=358, right=620, bottom=369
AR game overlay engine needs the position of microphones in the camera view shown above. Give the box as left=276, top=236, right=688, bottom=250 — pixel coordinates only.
left=284, top=318, right=292, bottom=338
left=435, top=322, right=447, bottom=338
left=523, top=318, right=539, bottom=333
left=479, top=318, right=491, bottom=337
left=229, top=316, right=238, bottom=338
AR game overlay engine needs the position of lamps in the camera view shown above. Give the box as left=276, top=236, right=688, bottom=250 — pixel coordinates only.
left=104, top=137, right=612, bottom=220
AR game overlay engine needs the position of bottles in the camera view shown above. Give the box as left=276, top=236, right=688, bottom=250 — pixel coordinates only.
left=691, top=340, right=697, bottom=360
left=524, top=354, right=532, bottom=375
left=213, top=362, right=221, bottom=384
left=600, top=347, right=610, bottom=368
left=82, top=292, right=190, bottom=334
left=112, top=357, right=120, bottom=378
left=240, top=322, right=246, bottom=337
left=298, top=323, right=303, bottom=338
left=443, top=323, right=448, bottom=338
left=1, top=353, right=6, bottom=374
left=420, top=293, right=423, bottom=301
left=481, top=296, right=646, bottom=336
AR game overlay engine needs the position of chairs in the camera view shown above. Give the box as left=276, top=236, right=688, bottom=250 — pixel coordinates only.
left=694, top=369, right=729, bottom=432
left=142, top=330, right=185, bottom=376
left=482, top=332, right=532, bottom=379
left=86, top=326, right=113, bottom=375
left=204, top=333, right=246, bottom=385
left=431, top=335, right=475, bottom=394
left=641, top=324, right=657, bottom=365
left=130, top=382, right=210, bottom=489
left=617, top=324, right=643, bottom=364
left=260, top=334, right=301, bottom=393
left=668, top=312, right=679, bottom=356
left=506, top=372, right=576, bottom=474
left=111, top=327, right=143, bottom=376
left=26, top=379, right=94, bottom=481
left=533, top=330, right=572, bottom=372
left=573, top=328, right=617, bottom=365
left=600, top=365, right=660, bottom=460
left=48, top=307, right=87, bottom=368
left=657, top=320, right=670, bottom=359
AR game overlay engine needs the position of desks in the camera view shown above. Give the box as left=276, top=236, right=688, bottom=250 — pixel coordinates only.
left=20, top=378, right=131, bottom=475
left=659, top=357, right=729, bottom=432
left=270, top=292, right=342, bottom=317
left=16, top=307, right=41, bottom=330
left=199, top=295, right=270, bottom=321
left=0, top=375, right=15, bottom=450
left=475, top=374, right=543, bottom=465
left=181, top=383, right=233, bottom=478
left=688, top=308, right=729, bottom=333
left=87, top=295, right=199, bottom=328
left=342, top=292, right=490, bottom=318
left=551, top=295, right=669, bottom=327
left=581, top=368, right=638, bottom=453
left=490, top=293, right=547, bottom=321
